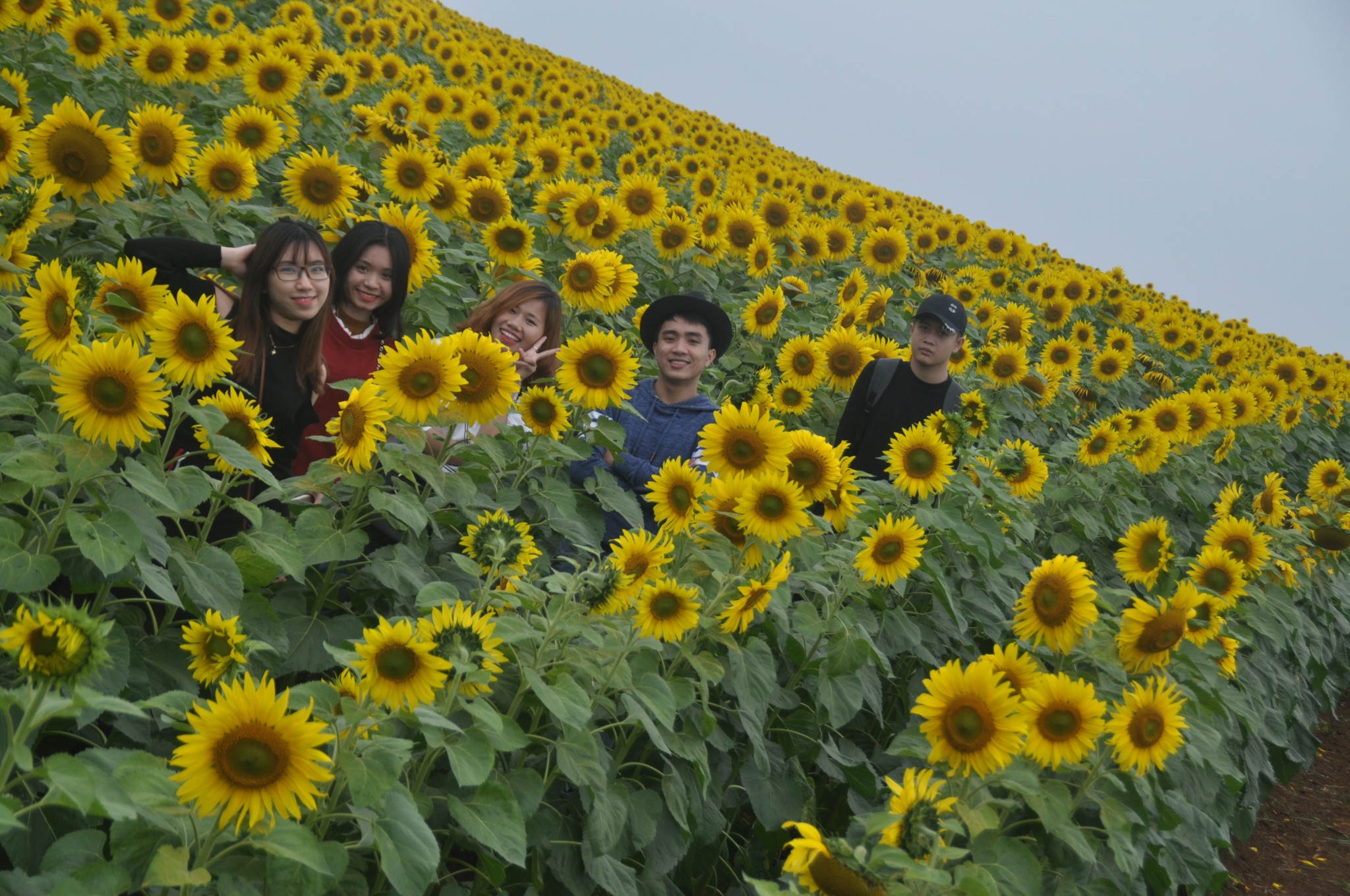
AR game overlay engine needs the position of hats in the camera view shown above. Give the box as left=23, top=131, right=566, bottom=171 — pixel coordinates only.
left=910, top=294, right=967, bottom=336
left=640, top=290, right=732, bottom=361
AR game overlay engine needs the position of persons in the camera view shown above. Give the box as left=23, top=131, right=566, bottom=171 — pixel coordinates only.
left=285, top=219, right=409, bottom=507
left=410, top=277, right=560, bottom=480
left=540, top=291, right=737, bottom=575
left=808, top=294, right=971, bottom=533
left=130, top=218, right=341, bottom=617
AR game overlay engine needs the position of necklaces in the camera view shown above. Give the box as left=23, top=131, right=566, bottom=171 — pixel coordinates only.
left=269, top=335, right=299, bottom=356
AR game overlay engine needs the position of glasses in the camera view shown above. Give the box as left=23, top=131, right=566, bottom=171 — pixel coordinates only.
left=269, top=262, right=331, bottom=281
